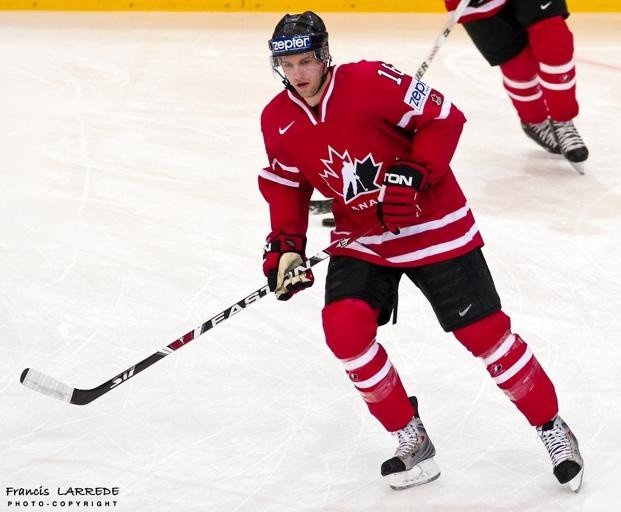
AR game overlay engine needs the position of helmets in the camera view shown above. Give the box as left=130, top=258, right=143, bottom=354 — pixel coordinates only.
left=269, top=11, right=330, bottom=66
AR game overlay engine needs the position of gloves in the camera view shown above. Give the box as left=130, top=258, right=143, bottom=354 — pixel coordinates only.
left=262, top=230, right=314, bottom=301
left=378, top=161, right=429, bottom=234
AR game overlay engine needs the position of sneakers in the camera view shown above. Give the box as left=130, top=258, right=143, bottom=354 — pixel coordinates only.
left=550, top=118, right=588, bottom=162
left=521, top=117, right=561, bottom=154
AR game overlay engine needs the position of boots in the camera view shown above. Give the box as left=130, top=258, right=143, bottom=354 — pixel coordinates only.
left=536, top=415, right=583, bottom=484
left=381, top=396, right=435, bottom=476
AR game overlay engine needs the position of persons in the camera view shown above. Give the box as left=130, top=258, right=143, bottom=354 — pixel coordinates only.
left=258, top=10, right=585, bottom=485
left=443, top=0, right=591, bottom=163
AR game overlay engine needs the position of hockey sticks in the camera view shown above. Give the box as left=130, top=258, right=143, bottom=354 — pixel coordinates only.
left=309, top=0, right=472, bottom=213
left=20, top=227, right=370, bottom=405
